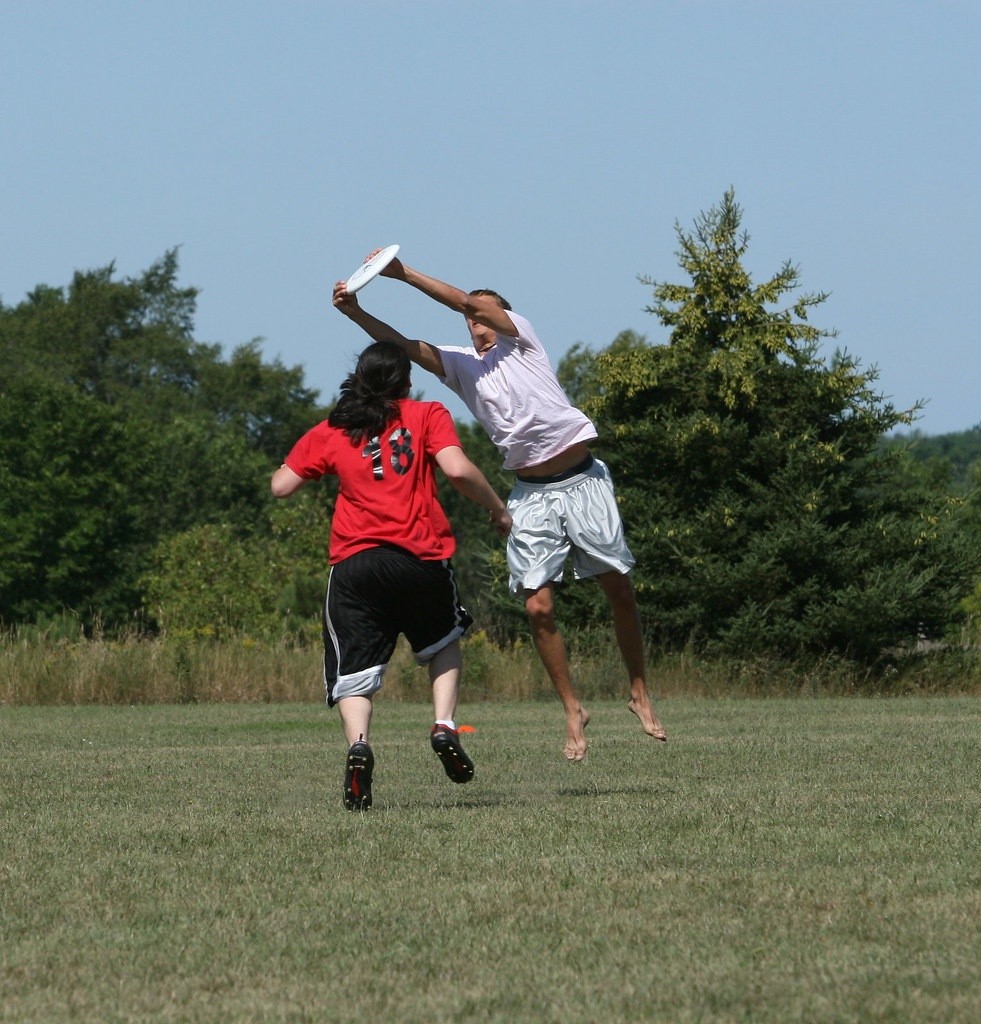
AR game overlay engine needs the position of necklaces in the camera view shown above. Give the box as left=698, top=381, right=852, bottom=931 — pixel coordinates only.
left=474, top=342, right=496, bottom=352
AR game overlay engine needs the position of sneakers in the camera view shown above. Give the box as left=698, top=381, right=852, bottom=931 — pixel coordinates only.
left=429, top=724, right=475, bottom=785
left=342, top=734, right=374, bottom=810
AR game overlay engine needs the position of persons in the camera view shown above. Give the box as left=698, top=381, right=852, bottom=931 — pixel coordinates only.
left=332, top=248, right=666, bottom=762
left=271, top=342, right=512, bottom=812
